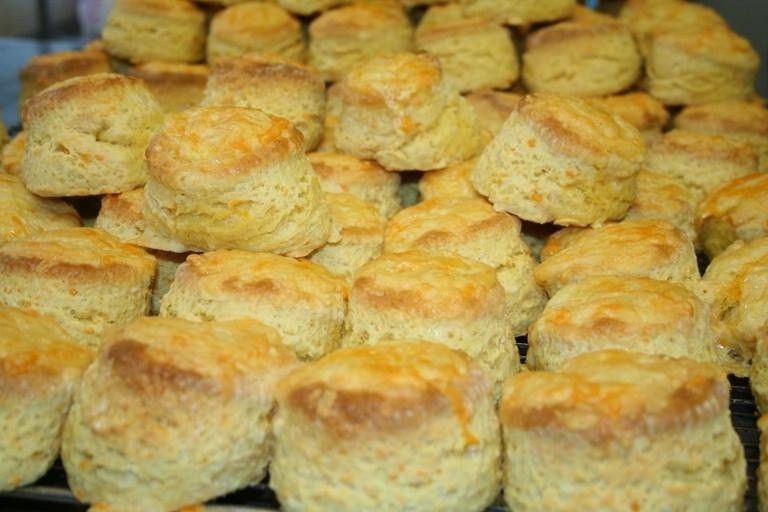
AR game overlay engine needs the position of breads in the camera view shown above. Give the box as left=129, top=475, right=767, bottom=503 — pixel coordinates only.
left=1, top=1, right=768, bottom=512
